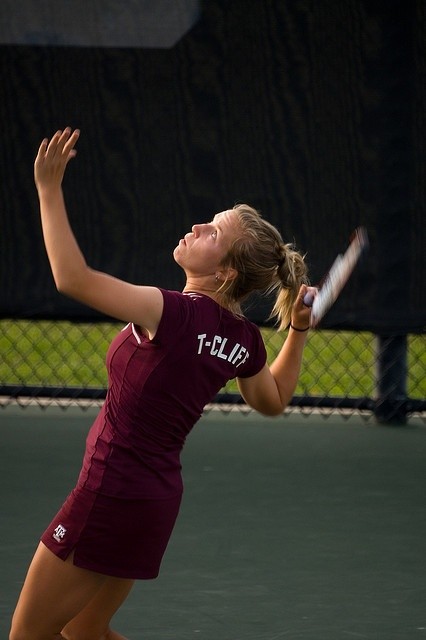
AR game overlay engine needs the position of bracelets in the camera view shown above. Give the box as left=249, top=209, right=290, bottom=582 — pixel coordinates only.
left=290, top=324, right=309, bottom=332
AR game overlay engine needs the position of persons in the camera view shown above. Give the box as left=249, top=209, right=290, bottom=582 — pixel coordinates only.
left=9, top=127, right=319, bottom=639
